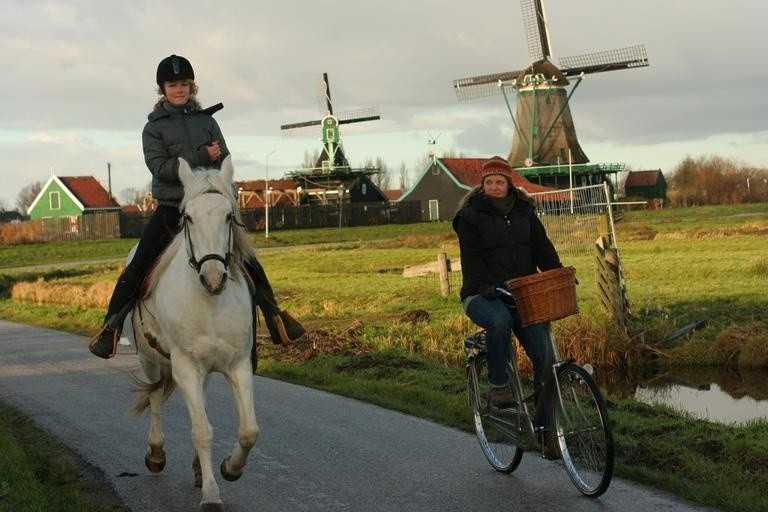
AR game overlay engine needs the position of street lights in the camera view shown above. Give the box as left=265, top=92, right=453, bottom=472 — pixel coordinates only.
left=265, top=149, right=278, bottom=238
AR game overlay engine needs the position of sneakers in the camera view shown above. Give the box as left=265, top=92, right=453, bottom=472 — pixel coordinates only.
left=538, top=429, right=562, bottom=461
left=490, top=387, right=513, bottom=406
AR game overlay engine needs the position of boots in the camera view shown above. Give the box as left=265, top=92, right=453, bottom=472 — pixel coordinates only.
left=90, top=267, right=140, bottom=359
left=246, top=259, right=290, bottom=344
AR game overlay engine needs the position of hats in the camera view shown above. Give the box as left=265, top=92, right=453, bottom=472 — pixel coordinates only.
left=480, top=156, right=513, bottom=181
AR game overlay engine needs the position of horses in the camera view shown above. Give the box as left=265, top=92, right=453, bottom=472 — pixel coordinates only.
left=122, top=154, right=260, bottom=512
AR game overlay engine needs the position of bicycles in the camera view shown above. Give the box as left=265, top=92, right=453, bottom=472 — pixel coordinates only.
left=464, top=288, right=613, bottom=497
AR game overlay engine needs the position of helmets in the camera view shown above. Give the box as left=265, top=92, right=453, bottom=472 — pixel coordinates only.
left=156, top=55, right=194, bottom=86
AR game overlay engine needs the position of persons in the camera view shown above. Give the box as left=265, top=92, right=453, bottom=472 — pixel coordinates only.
left=452, top=157, right=580, bottom=459
left=88, top=55, right=306, bottom=359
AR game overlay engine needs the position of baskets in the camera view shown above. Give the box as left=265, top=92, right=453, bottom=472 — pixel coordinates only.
left=509, top=266, right=580, bottom=329
